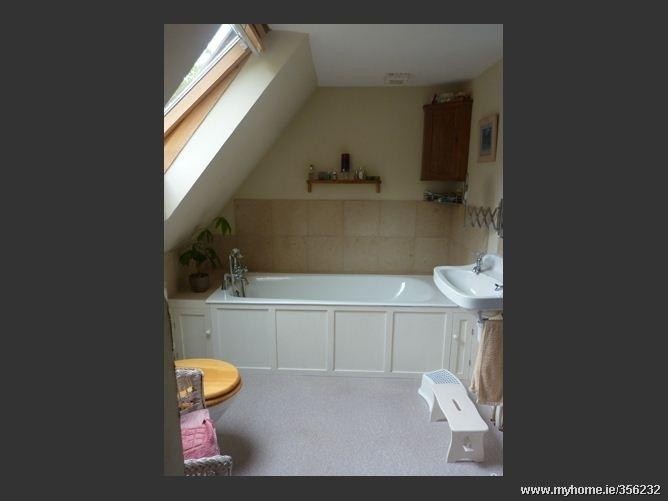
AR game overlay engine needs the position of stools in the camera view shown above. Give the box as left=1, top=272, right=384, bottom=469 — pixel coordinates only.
left=418, top=368, right=490, bottom=464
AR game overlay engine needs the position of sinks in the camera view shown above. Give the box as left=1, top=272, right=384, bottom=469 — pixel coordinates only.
left=433, top=265, right=503, bottom=311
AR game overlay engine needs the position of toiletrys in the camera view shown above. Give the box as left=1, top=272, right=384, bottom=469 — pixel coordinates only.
left=307, top=164, right=368, bottom=182
left=340, top=154, right=350, bottom=173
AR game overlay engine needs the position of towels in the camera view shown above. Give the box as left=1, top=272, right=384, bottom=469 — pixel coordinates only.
left=468, top=319, right=503, bottom=406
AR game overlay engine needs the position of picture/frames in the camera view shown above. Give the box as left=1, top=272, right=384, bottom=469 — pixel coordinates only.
left=477, top=113, right=499, bottom=162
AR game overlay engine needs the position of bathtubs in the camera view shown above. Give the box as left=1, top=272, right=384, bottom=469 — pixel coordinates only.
left=205, top=273, right=459, bottom=309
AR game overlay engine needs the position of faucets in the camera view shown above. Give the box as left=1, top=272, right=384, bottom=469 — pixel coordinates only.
left=223, top=274, right=249, bottom=292
left=472, top=258, right=481, bottom=273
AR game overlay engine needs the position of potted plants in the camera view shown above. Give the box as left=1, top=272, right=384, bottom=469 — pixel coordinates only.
left=180, top=217, right=232, bottom=293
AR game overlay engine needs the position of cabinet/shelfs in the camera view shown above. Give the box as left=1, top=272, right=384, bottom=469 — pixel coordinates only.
left=168, top=308, right=213, bottom=360
left=448, top=313, right=481, bottom=381
